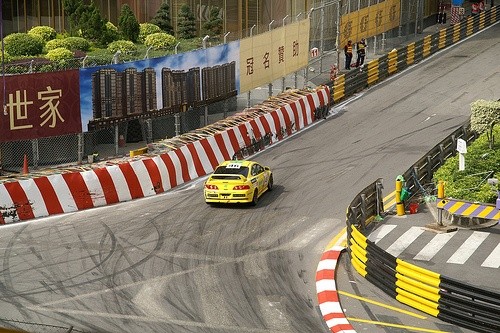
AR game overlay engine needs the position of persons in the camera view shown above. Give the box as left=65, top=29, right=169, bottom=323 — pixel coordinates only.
left=471, top=0, right=479, bottom=17
left=479, top=0, right=485, bottom=13
left=344, top=40, right=353, bottom=70
left=356, top=38, right=367, bottom=68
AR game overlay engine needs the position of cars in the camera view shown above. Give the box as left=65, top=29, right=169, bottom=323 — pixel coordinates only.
left=203, top=160, right=274, bottom=207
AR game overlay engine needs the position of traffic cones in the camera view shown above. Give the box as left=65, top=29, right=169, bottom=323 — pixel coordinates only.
left=22, top=154, right=29, bottom=174
